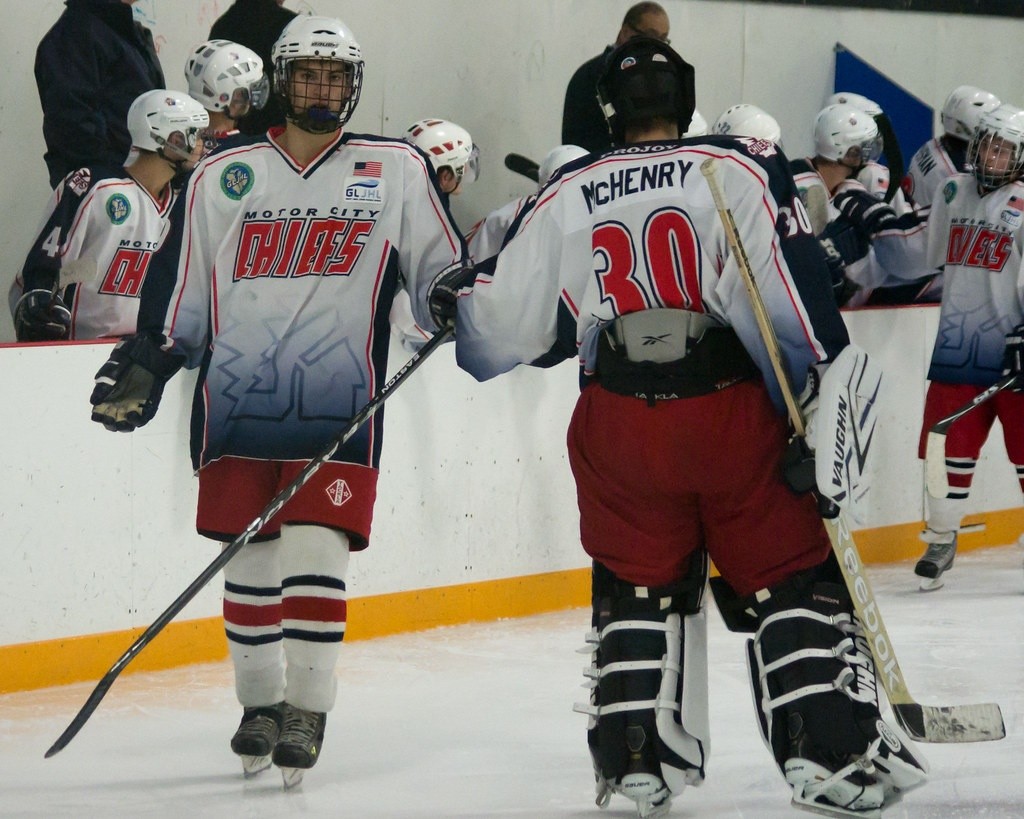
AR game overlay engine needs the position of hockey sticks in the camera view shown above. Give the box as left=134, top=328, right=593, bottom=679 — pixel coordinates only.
left=41, top=325, right=459, bottom=763
left=503, top=151, right=542, bottom=184
left=42, top=256, right=100, bottom=340
left=807, top=183, right=829, bottom=238
left=838, top=111, right=909, bottom=309
left=699, top=151, right=1013, bottom=749
left=922, top=370, right=1018, bottom=502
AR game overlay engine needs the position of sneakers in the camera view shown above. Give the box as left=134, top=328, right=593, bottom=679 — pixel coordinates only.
left=913, top=528, right=958, bottom=592
left=595, top=680, right=687, bottom=819
left=231, top=701, right=285, bottom=781
left=270, top=703, right=329, bottom=792
left=777, top=670, right=904, bottom=819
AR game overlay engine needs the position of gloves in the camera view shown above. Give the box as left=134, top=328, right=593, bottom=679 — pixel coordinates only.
left=1000, top=325, right=1024, bottom=397
left=12, top=289, right=73, bottom=343
left=427, top=265, right=474, bottom=330
left=89, top=334, right=182, bottom=433
left=777, top=343, right=888, bottom=535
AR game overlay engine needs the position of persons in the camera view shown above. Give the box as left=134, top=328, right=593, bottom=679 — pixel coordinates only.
left=401, top=118, right=481, bottom=196
left=7, top=88, right=210, bottom=342
left=183, top=39, right=269, bottom=160
left=537, top=0, right=1001, bottom=308
left=90, top=13, right=473, bottom=788
left=832, top=102, right=1024, bottom=592
left=207, top=0, right=300, bottom=133
left=33, top=1, right=167, bottom=192
left=455, top=39, right=926, bottom=819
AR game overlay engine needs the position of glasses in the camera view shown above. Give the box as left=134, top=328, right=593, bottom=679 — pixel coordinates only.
left=626, top=20, right=671, bottom=45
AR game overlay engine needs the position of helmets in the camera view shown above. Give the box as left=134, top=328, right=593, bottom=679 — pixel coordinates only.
left=401, top=119, right=481, bottom=184
left=972, top=102, right=1024, bottom=191
left=595, top=34, right=696, bottom=148
left=823, top=91, right=884, bottom=121
left=269, top=11, right=369, bottom=134
left=939, top=85, right=1002, bottom=142
left=537, top=144, right=591, bottom=190
left=184, top=39, right=270, bottom=113
left=127, top=88, right=211, bottom=155
left=681, top=109, right=709, bottom=139
left=711, top=103, right=781, bottom=145
left=813, top=102, right=883, bottom=165
